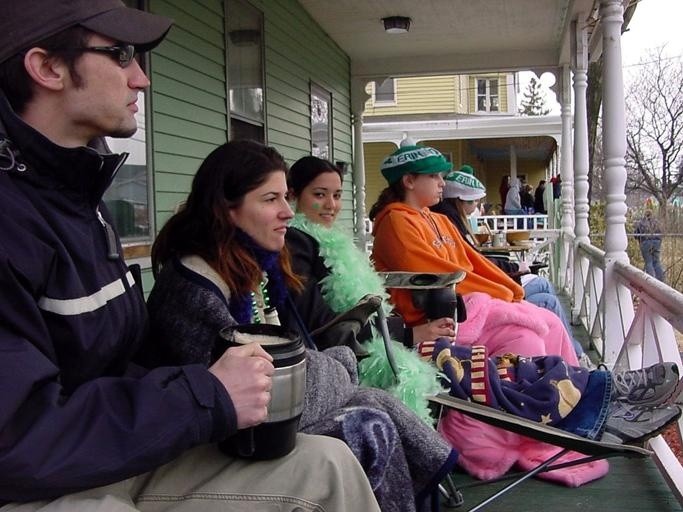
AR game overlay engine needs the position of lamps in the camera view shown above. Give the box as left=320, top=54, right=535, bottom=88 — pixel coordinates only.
left=378, top=15, right=413, bottom=34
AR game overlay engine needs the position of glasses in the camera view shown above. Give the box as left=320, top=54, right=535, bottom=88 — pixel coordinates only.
left=20, top=43, right=136, bottom=69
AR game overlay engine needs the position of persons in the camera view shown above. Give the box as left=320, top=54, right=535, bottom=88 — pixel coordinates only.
left=369, top=142, right=525, bottom=327
left=145, top=137, right=461, bottom=510
left=497, top=171, right=563, bottom=216
left=633, top=208, right=666, bottom=284
left=1, top=1, right=382, bottom=512
left=284, top=154, right=683, bottom=446
left=443, top=161, right=600, bottom=373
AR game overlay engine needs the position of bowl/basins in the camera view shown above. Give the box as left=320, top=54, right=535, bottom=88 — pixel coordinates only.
left=505, top=230, right=531, bottom=246
left=474, top=233, right=488, bottom=244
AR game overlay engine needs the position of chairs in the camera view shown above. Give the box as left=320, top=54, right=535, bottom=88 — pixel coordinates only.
left=505, top=231, right=532, bottom=248
left=474, top=233, right=491, bottom=247
left=276, top=265, right=656, bottom=511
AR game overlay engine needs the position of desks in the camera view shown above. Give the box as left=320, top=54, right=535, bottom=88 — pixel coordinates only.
left=477, top=240, right=532, bottom=263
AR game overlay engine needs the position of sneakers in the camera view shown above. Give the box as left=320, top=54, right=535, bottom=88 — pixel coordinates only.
left=600, top=397, right=682, bottom=445
left=611, top=362, right=679, bottom=407
left=579, top=355, right=598, bottom=372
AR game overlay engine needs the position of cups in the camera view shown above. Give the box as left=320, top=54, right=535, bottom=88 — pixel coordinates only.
left=491, top=234, right=503, bottom=247
left=207, top=322, right=307, bottom=461
left=439, top=322, right=459, bottom=348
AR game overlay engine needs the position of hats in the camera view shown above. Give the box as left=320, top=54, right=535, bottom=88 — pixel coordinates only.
left=381, top=145, right=453, bottom=184
left=443, top=171, right=487, bottom=201
left=0, top=0, right=173, bottom=64
